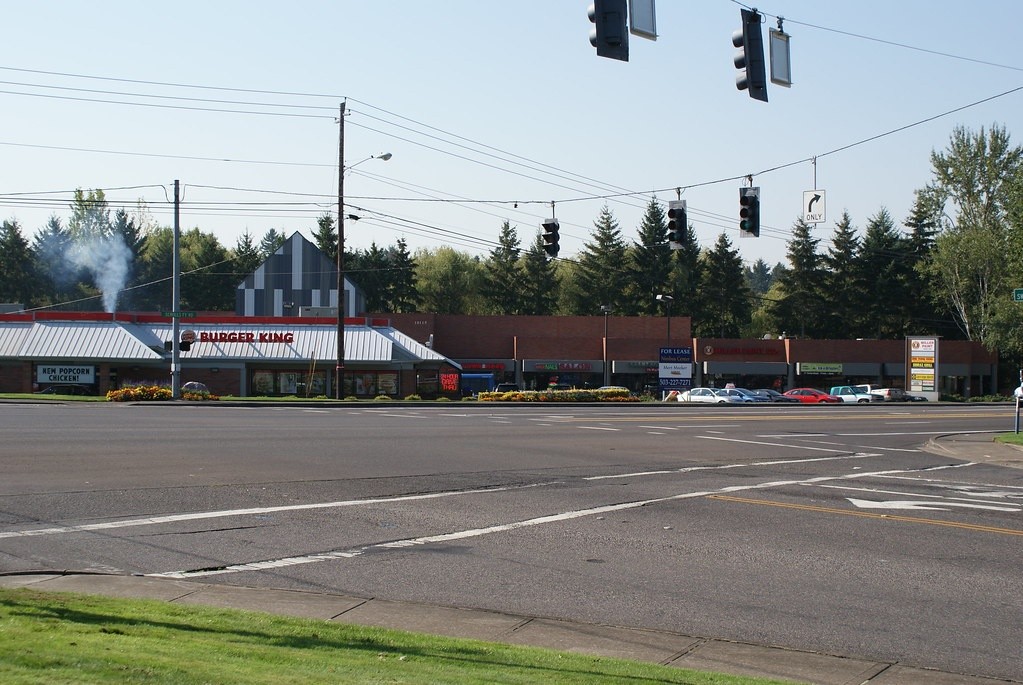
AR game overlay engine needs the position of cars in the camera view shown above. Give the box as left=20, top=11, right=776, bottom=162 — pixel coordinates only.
left=547, top=384, right=572, bottom=392
left=706, top=388, right=771, bottom=403
left=782, top=388, right=842, bottom=404
left=903, top=390, right=928, bottom=402
left=599, top=386, right=629, bottom=391
left=495, top=383, right=520, bottom=394
left=677, top=387, right=736, bottom=404
left=750, top=389, right=799, bottom=403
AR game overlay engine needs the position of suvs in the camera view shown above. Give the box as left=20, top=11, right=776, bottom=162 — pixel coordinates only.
left=830, top=386, right=884, bottom=404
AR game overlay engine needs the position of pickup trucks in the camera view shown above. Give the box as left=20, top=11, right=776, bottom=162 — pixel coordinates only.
left=853, top=384, right=903, bottom=401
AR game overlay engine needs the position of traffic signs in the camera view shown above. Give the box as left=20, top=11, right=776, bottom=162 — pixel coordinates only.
left=803, top=189, right=826, bottom=224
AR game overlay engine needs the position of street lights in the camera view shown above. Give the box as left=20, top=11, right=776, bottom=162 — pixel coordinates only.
left=334, top=103, right=392, bottom=401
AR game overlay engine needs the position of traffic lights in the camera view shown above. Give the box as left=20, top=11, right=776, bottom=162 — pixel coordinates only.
left=731, top=9, right=768, bottom=103
left=542, top=218, right=561, bottom=258
left=739, top=187, right=760, bottom=238
left=668, top=200, right=688, bottom=250
